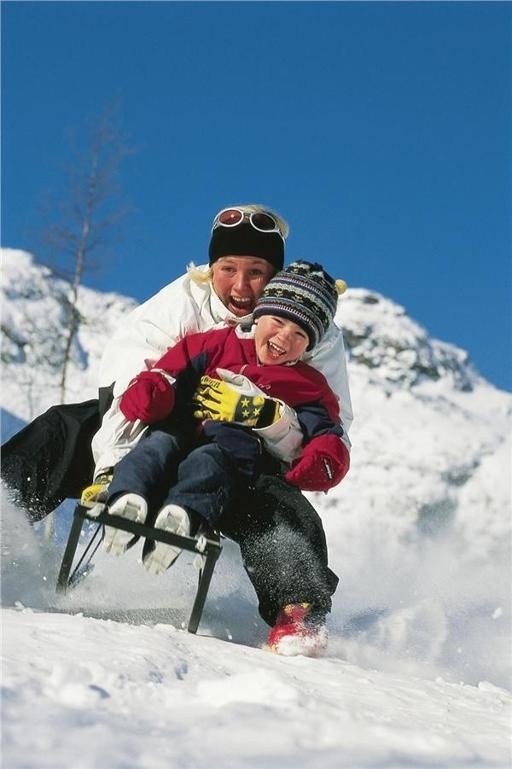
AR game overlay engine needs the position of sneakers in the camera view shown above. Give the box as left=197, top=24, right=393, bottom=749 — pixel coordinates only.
left=103, top=493, right=148, bottom=557
left=143, top=505, right=191, bottom=574
left=267, top=603, right=327, bottom=654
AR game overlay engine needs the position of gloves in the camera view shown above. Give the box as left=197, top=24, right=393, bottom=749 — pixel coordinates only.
left=80, top=474, right=114, bottom=504
left=192, top=370, right=282, bottom=427
left=119, top=372, right=176, bottom=424
left=286, top=434, right=353, bottom=490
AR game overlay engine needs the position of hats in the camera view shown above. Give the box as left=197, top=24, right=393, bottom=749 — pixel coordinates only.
left=210, top=204, right=289, bottom=269
left=253, top=260, right=337, bottom=350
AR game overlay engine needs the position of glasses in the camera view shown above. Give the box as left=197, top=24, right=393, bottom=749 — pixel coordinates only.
left=212, top=210, right=285, bottom=240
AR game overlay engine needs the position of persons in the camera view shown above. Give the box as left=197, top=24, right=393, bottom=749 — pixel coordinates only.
left=106, top=260, right=350, bottom=579
left=0, top=202, right=354, bottom=657
